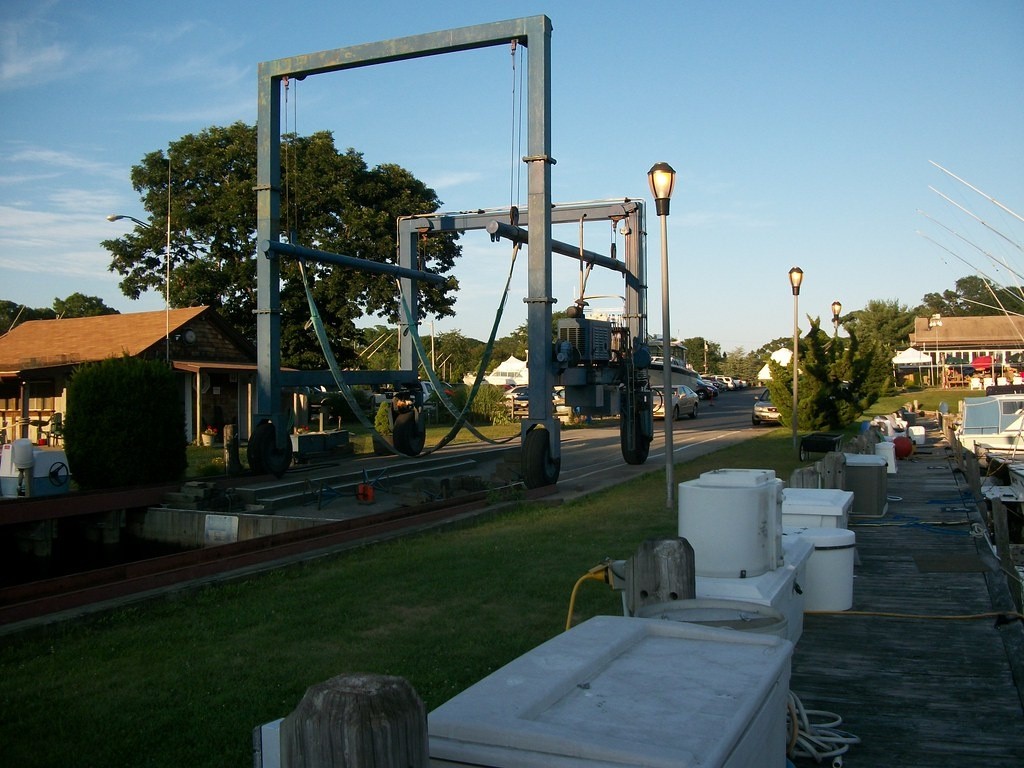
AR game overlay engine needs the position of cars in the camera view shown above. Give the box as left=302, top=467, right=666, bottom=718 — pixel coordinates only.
left=651, top=356, right=750, bottom=397
left=646, top=386, right=699, bottom=420
left=752, top=386, right=785, bottom=426
left=422, top=375, right=568, bottom=412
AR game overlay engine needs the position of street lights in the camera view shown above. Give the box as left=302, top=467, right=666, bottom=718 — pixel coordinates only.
left=648, top=161, right=676, bottom=508
left=789, top=266, right=804, bottom=465
left=831, top=301, right=843, bottom=340
